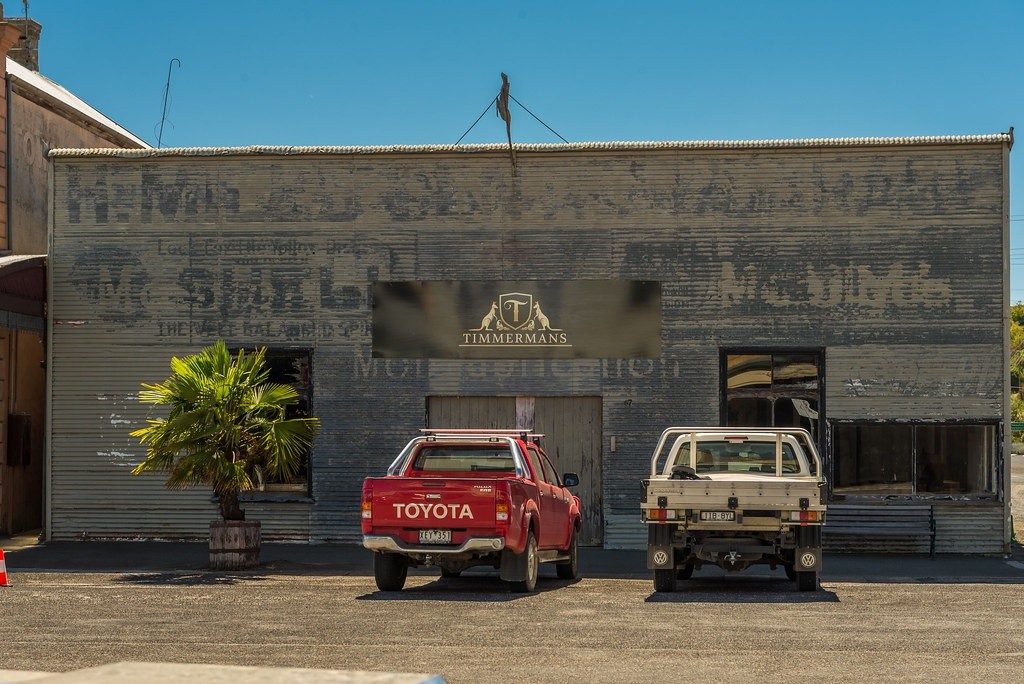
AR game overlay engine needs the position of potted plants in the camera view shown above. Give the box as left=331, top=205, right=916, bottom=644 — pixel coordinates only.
left=126, top=341, right=321, bottom=570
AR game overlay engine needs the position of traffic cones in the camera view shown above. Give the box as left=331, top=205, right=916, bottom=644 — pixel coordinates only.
left=0, top=549, right=13, bottom=587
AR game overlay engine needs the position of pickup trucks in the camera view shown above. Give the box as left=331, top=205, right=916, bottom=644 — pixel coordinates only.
left=360, top=427, right=582, bottom=593
left=640, top=426, right=828, bottom=592
